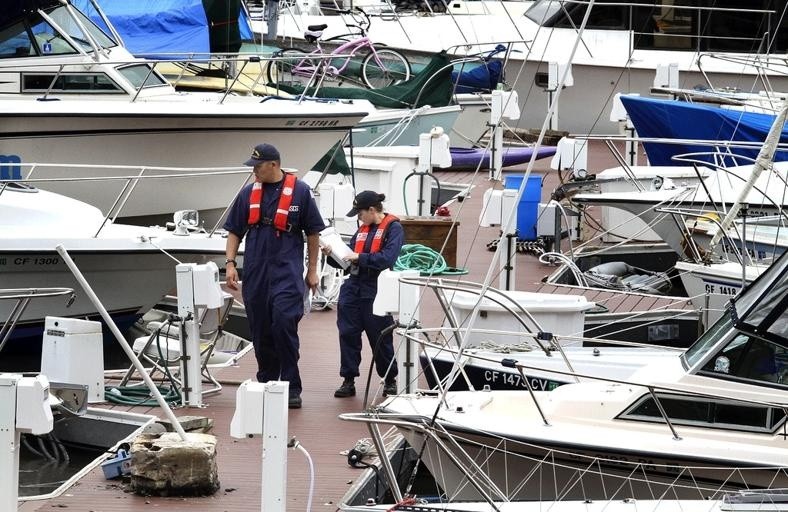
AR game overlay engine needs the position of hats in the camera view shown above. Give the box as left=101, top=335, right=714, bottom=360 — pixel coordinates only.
left=346, top=191, right=380, bottom=217
left=243, top=143, right=280, bottom=166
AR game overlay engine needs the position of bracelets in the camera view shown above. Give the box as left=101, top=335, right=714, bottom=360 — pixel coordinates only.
left=224, top=257, right=237, bottom=269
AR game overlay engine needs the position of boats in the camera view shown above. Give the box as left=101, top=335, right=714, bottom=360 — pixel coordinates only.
left=336, top=246, right=788, bottom=512
left=0, top=0, right=788, bottom=390
left=0, top=161, right=299, bottom=369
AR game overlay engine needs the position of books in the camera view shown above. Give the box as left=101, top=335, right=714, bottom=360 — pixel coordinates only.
left=316, top=225, right=355, bottom=271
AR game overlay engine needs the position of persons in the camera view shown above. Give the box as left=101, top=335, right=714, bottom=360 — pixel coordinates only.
left=317, top=189, right=404, bottom=399
left=221, top=142, right=326, bottom=410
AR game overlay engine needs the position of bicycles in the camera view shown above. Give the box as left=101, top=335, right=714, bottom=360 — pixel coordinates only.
left=267, top=9, right=413, bottom=97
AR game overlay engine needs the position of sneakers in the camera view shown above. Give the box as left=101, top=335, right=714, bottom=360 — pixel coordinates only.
left=335, top=378, right=357, bottom=397
left=383, top=381, right=397, bottom=397
left=288, top=389, right=302, bottom=408
